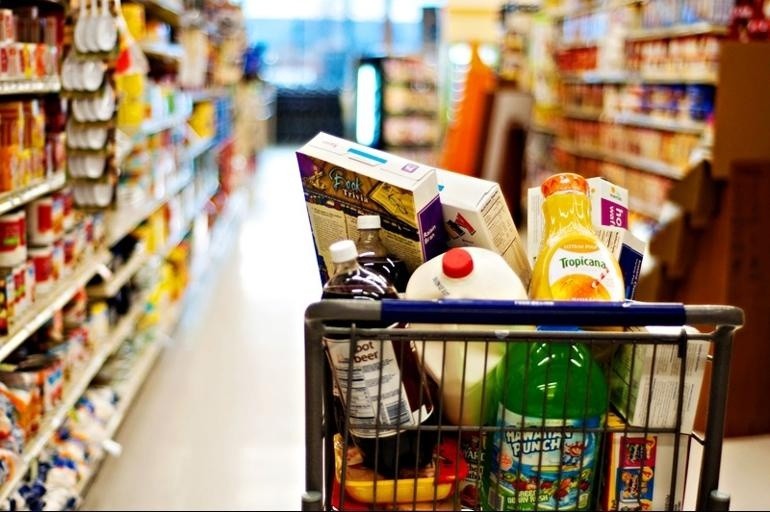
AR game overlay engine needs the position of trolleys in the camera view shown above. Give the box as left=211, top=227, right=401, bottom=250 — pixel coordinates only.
left=297, top=296, right=750, bottom=512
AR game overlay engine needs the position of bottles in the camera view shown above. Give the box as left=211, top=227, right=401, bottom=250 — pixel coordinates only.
left=478, top=321, right=603, bottom=510
left=315, top=240, right=444, bottom=474
left=530, top=175, right=627, bottom=358
left=353, top=214, right=408, bottom=299
